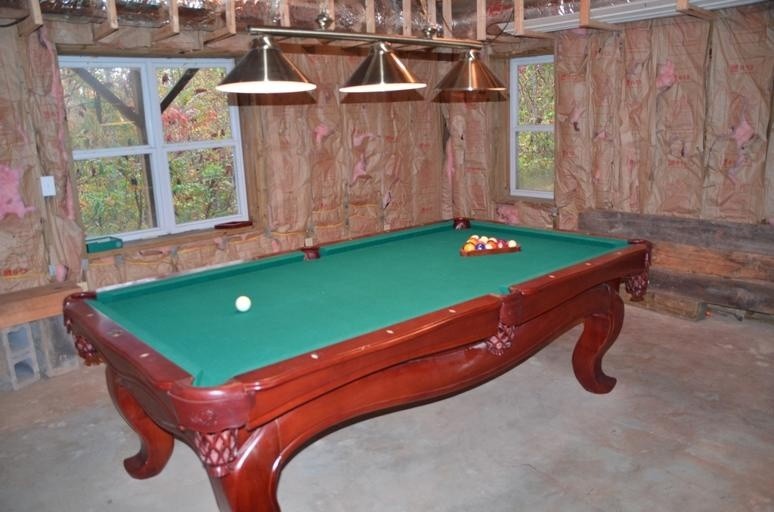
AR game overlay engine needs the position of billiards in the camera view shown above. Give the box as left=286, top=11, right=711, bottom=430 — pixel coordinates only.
left=463, top=233, right=517, bottom=252
left=235, top=295, right=251, bottom=311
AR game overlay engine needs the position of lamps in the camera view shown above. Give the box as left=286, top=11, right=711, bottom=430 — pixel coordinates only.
left=213, top=7, right=506, bottom=97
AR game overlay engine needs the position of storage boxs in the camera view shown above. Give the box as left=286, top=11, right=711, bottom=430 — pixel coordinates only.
left=0, top=278, right=83, bottom=330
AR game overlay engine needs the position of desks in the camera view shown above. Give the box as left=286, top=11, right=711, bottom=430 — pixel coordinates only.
left=62, top=215, right=656, bottom=511
